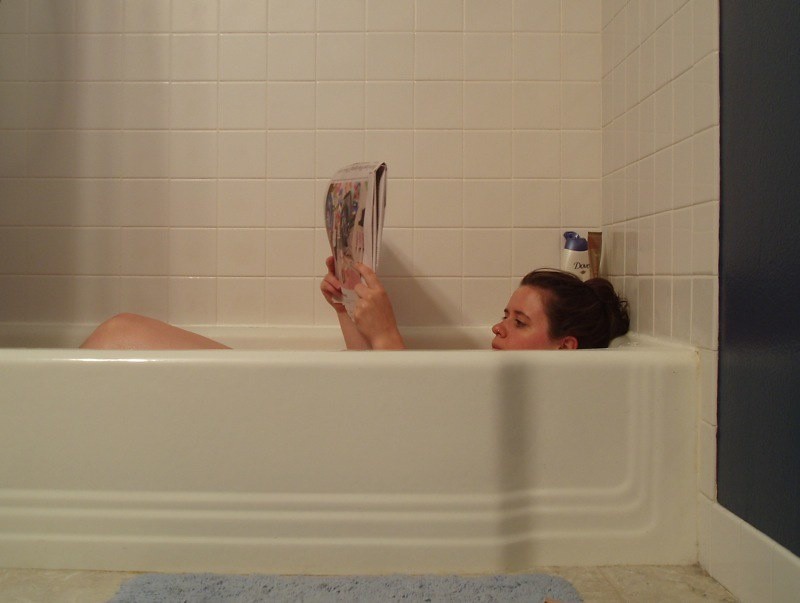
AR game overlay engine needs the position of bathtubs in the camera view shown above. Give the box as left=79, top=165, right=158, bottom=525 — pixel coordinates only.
left=3, top=322, right=703, bottom=574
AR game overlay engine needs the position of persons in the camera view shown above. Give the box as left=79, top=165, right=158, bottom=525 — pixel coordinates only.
left=78, top=255, right=632, bottom=350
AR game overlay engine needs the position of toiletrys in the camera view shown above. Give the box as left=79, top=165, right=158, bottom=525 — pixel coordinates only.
left=563, top=230, right=590, bottom=283
left=588, top=232, right=602, bottom=280
